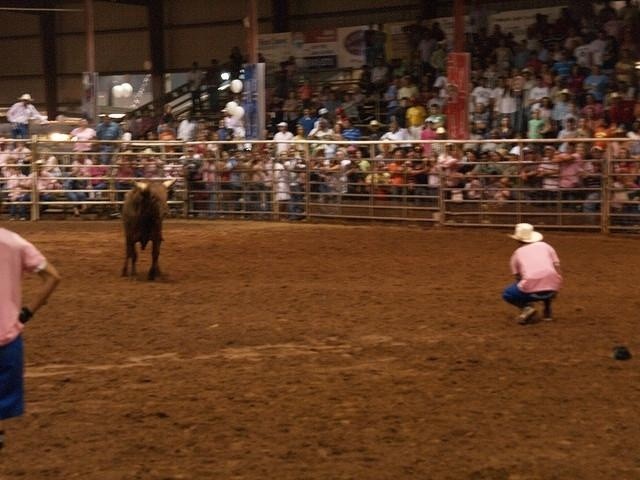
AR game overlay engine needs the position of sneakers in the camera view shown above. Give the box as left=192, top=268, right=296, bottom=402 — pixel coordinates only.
left=516, top=307, right=537, bottom=325
left=538, top=315, right=553, bottom=321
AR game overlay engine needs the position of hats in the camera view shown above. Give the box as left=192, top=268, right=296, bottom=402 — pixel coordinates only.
left=1, top=23, right=637, bottom=169
left=507, top=223, right=544, bottom=243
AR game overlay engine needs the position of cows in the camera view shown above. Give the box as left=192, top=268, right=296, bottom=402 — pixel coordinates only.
left=121, top=177, right=177, bottom=280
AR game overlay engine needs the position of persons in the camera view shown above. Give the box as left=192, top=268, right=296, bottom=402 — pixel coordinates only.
left=0, top=225, right=60, bottom=448
left=501, top=223, right=564, bottom=324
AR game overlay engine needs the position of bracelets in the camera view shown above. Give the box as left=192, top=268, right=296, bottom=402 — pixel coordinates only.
left=20, top=305, right=32, bottom=324
